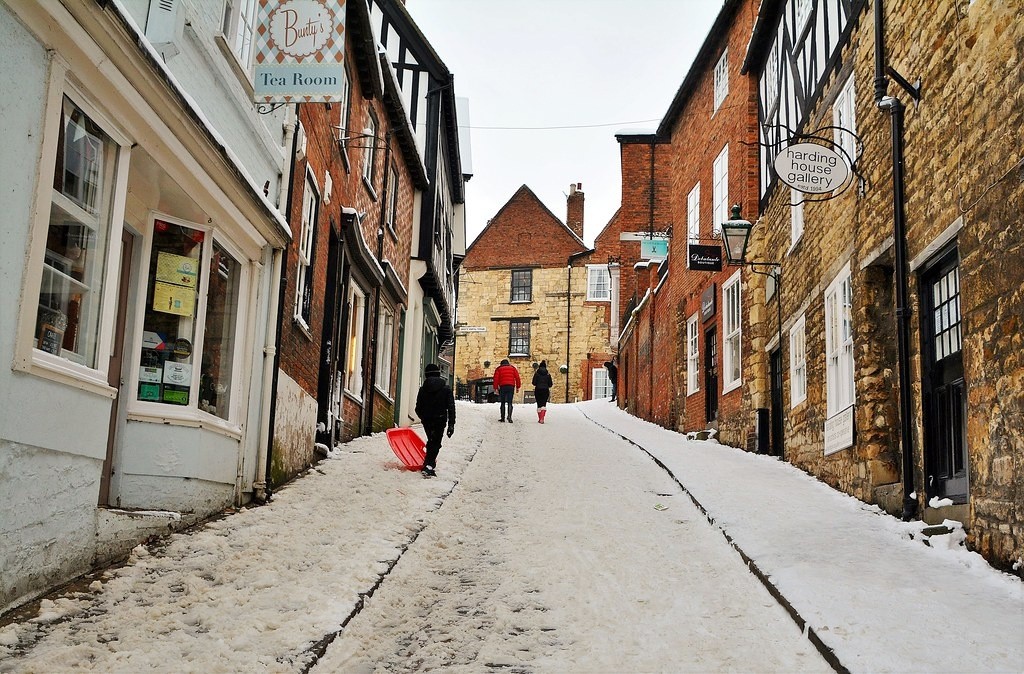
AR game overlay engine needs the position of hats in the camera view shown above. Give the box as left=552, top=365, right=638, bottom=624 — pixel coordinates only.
left=501, top=360, right=509, bottom=365
left=540, top=360, right=546, bottom=368
left=425, top=364, right=440, bottom=377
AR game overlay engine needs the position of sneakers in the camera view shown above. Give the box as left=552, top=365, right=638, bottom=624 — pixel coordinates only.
left=421, top=466, right=437, bottom=479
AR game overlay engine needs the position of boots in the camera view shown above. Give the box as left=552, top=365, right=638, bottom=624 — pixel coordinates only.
left=507, top=406, right=513, bottom=423
left=609, top=395, right=616, bottom=402
left=498, top=406, right=505, bottom=422
left=537, top=406, right=547, bottom=423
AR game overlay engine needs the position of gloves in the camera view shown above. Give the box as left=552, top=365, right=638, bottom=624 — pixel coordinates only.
left=447, top=425, right=454, bottom=438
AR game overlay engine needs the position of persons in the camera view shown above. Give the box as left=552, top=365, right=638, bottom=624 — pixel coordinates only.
left=415, top=363, right=455, bottom=477
left=604, top=355, right=617, bottom=402
left=493, top=359, right=521, bottom=423
left=532, top=360, right=553, bottom=424
